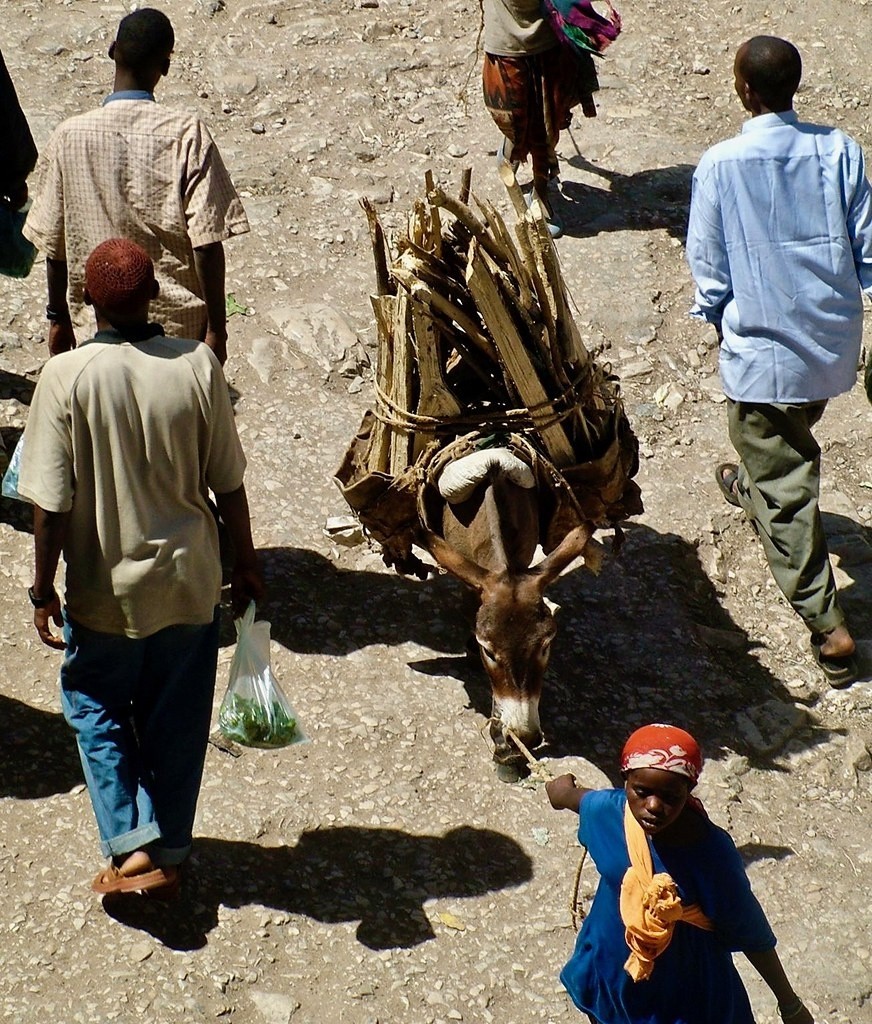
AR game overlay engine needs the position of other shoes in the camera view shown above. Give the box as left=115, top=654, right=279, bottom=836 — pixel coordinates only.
left=497, top=137, right=521, bottom=182
left=529, top=184, right=565, bottom=239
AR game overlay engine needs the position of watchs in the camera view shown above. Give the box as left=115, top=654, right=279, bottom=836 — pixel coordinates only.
left=27, top=584, right=55, bottom=609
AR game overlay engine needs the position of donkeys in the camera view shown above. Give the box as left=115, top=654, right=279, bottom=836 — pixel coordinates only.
left=409, top=459, right=598, bottom=751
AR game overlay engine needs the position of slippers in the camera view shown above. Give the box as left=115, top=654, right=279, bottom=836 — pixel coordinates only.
left=810, top=630, right=862, bottom=686
left=93, top=858, right=182, bottom=895
left=712, top=462, right=762, bottom=538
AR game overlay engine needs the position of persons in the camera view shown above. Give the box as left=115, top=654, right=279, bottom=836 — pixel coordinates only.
left=20, top=5, right=252, bottom=369
left=479, top=2, right=622, bottom=240
left=15, top=238, right=266, bottom=908
left=0, top=50, right=39, bottom=271
left=685, top=32, right=872, bottom=689
left=543, top=722, right=816, bottom=1024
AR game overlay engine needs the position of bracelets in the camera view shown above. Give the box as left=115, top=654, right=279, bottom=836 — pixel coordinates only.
left=776, top=993, right=804, bottom=1020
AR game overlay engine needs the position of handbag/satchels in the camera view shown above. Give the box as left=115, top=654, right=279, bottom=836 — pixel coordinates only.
left=217, top=605, right=311, bottom=755
